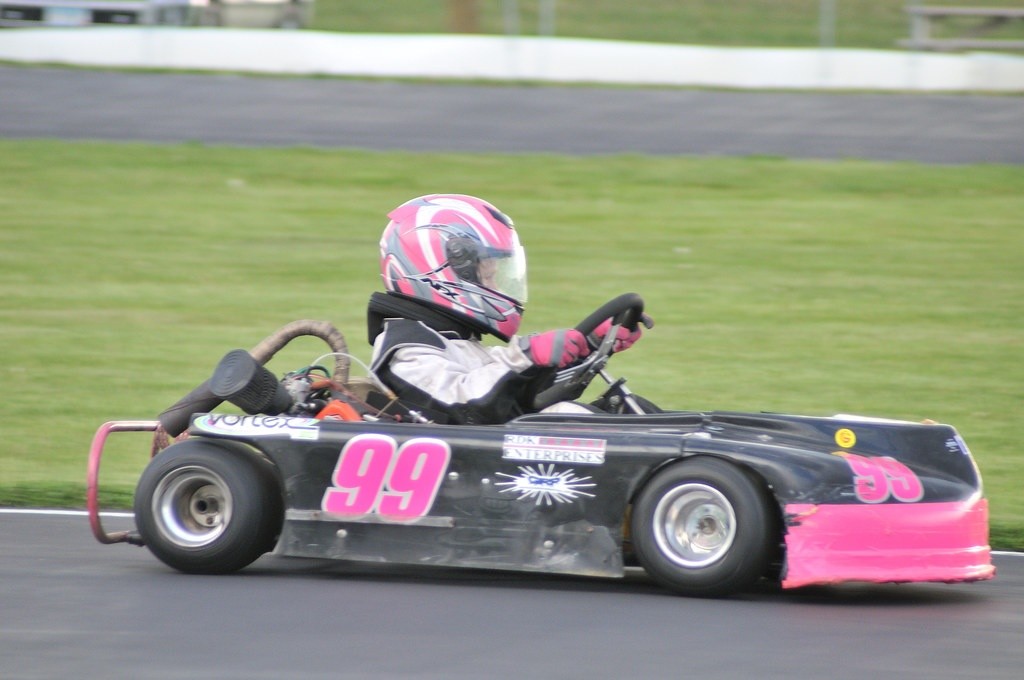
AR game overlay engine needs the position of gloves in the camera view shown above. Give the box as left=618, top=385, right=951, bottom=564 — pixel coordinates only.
left=521, top=329, right=590, bottom=368
left=587, top=311, right=655, bottom=355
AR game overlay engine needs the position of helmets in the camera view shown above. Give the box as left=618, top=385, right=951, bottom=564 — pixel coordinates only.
left=379, top=193, right=528, bottom=342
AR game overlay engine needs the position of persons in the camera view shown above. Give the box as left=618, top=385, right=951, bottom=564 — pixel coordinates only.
left=365, top=193, right=664, bottom=426
